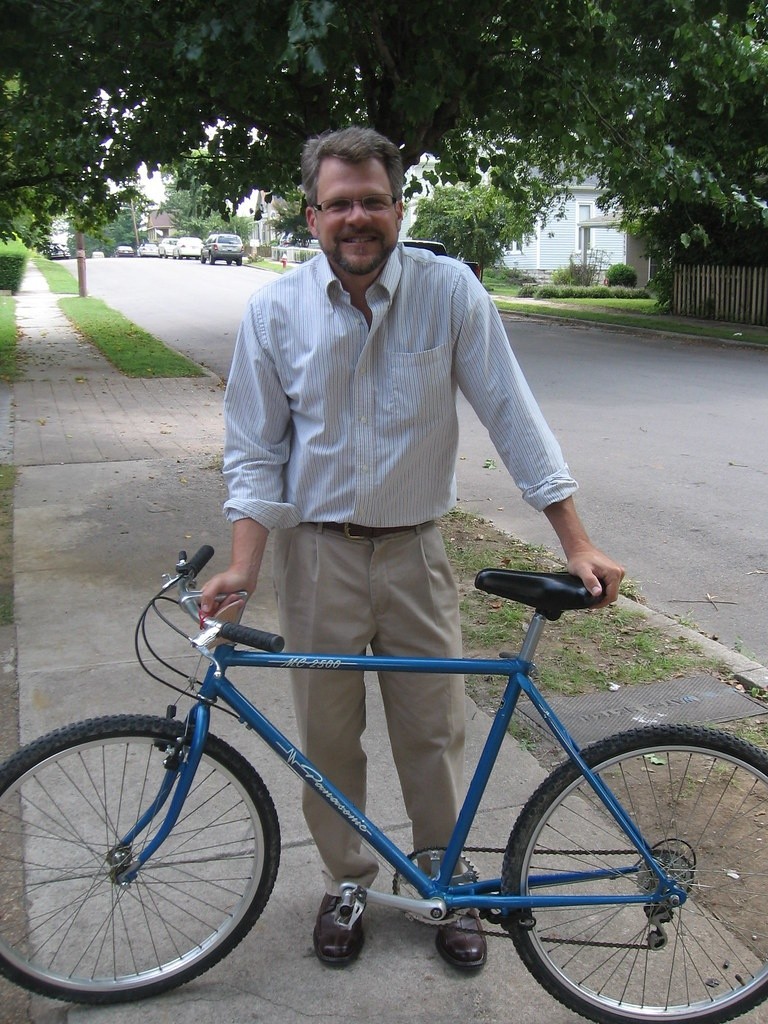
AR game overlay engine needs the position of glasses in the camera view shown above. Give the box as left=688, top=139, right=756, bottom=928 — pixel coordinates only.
left=313, top=194, right=397, bottom=218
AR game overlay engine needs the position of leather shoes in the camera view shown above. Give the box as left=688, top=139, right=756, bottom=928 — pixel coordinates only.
left=314, top=890, right=362, bottom=967
left=437, top=915, right=487, bottom=972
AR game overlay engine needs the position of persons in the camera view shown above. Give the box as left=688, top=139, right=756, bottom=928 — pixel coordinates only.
left=200, top=126, right=625, bottom=969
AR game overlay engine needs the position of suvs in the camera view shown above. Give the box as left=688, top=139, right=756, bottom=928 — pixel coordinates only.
left=200, top=234, right=244, bottom=267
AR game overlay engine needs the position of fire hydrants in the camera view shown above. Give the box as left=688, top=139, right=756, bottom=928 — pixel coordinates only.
left=280, top=253, right=288, bottom=268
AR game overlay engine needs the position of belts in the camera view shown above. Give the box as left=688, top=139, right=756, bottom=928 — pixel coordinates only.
left=309, top=521, right=417, bottom=539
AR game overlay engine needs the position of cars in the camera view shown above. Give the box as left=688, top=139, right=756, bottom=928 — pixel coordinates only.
left=45, top=242, right=72, bottom=260
left=113, top=246, right=135, bottom=257
left=136, top=243, right=160, bottom=257
left=158, top=237, right=180, bottom=259
left=173, top=237, right=204, bottom=260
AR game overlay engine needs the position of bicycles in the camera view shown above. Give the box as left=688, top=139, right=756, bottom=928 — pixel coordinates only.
left=0, top=544, right=768, bottom=1024
